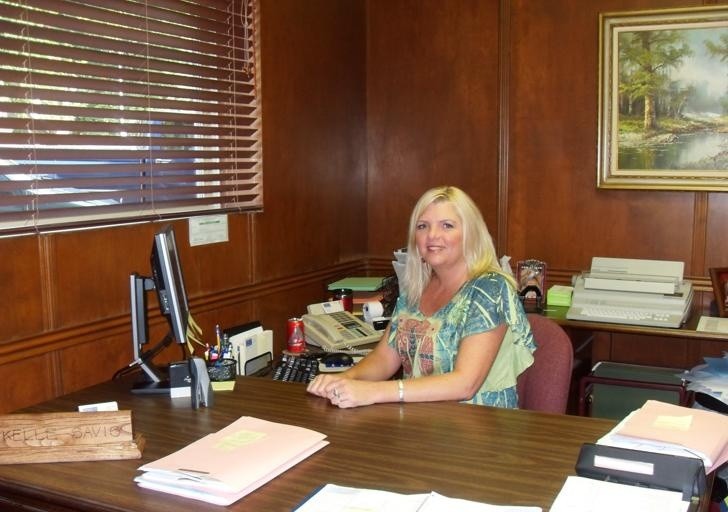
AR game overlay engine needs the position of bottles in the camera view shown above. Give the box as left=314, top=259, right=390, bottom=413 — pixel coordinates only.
left=333, top=288, right=353, bottom=315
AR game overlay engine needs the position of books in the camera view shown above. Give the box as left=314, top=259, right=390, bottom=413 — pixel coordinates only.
left=223, top=320, right=275, bottom=376
left=549, top=474, right=692, bottom=512
left=326, top=275, right=398, bottom=317
left=290, top=480, right=543, bottom=512
left=133, top=414, right=331, bottom=506
left=594, top=399, right=728, bottom=475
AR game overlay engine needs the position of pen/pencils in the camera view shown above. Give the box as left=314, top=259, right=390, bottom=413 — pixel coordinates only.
left=204, top=324, right=232, bottom=362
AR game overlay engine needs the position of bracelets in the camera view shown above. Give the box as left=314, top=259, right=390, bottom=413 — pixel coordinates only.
left=398, top=379, right=404, bottom=404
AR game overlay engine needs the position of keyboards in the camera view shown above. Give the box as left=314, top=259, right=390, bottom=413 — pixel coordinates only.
left=273, top=351, right=320, bottom=384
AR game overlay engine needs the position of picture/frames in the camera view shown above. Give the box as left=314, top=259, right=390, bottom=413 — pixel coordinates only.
left=708, top=266, right=728, bottom=317
left=595, top=5, right=728, bottom=192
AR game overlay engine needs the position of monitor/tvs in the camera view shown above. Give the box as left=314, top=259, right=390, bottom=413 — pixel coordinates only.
left=129, top=223, right=191, bottom=394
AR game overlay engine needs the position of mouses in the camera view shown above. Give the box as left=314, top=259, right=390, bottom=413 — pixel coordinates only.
left=321, top=352, right=353, bottom=366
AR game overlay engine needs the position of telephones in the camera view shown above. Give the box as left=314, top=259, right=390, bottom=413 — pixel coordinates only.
left=301, top=300, right=381, bottom=350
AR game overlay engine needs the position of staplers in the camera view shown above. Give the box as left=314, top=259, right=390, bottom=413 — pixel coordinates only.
left=189, top=358, right=214, bottom=409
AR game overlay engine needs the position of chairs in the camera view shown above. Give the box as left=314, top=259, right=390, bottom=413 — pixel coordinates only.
left=514, top=312, right=574, bottom=413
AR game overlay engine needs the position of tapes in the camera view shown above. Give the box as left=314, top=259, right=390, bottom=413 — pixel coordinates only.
left=362, top=301, right=387, bottom=324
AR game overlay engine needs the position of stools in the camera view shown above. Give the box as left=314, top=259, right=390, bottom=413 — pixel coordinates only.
left=576, top=360, right=697, bottom=416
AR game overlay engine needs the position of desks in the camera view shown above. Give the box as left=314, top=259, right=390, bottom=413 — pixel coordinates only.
left=523, top=282, right=728, bottom=417
left=0, top=363, right=728, bottom=512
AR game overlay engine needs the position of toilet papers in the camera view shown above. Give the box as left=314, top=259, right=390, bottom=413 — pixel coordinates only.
left=363, top=301, right=385, bottom=319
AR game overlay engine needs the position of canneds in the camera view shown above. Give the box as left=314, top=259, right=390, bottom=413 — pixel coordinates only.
left=287, top=318, right=304, bottom=352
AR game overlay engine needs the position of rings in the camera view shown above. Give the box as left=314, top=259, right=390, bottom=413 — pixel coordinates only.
left=333, top=388, right=338, bottom=397
left=338, top=394, right=343, bottom=402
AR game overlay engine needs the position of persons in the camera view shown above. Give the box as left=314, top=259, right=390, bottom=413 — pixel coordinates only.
left=307, top=184, right=541, bottom=411
